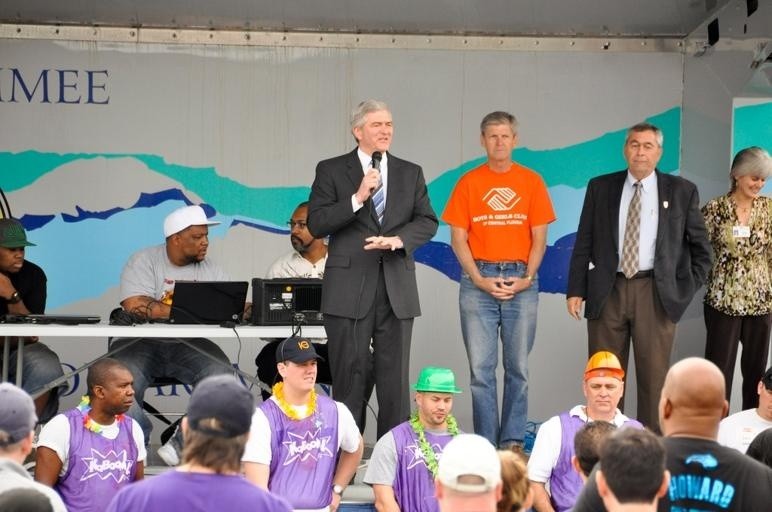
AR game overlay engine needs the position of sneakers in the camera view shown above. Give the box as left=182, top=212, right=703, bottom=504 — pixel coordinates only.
left=158, top=427, right=179, bottom=467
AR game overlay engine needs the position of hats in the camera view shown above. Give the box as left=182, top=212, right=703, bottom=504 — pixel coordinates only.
left=1, top=382, right=39, bottom=446
left=438, top=433, right=500, bottom=491
left=187, top=374, right=254, bottom=437
left=276, top=337, right=326, bottom=364
left=0, top=218, right=37, bottom=248
left=163, top=204, right=221, bottom=239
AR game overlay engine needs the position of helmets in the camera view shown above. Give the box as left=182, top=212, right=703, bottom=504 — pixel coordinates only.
left=413, top=366, right=464, bottom=394
left=585, top=351, right=621, bottom=372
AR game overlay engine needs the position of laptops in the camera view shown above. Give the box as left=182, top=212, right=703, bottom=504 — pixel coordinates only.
left=153, top=281, right=249, bottom=324
left=15, top=316, right=102, bottom=324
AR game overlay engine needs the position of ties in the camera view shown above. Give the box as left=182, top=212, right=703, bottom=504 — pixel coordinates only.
left=371, top=161, right=385, bottom=220
left=620, top=180, right=643, bottom=280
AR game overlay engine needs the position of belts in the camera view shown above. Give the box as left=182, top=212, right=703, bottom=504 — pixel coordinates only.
left=617, top=271, right=652, bottom=280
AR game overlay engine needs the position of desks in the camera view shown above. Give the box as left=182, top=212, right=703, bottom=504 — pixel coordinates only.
left=0, top=322, right=328, bottom=401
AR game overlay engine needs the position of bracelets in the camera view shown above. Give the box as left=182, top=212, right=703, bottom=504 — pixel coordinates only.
left=146, top=300, right=158, bottom=319
left=524, top=275, right=534, bottom=281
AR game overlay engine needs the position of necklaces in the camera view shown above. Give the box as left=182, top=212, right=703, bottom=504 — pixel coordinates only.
left=410, top=411, right=465, bottom=472
left=735, top=200, right=751, bottom=213
left=272, top=381, right=316, bottom=419
left=80, top=392, right=125, bottom=433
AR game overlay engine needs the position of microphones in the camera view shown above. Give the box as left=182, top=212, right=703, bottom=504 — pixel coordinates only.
left=370, top=151, right=382, bottom=193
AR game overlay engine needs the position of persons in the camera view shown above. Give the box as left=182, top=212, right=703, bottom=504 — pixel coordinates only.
left=103, top=204, right=246, bottom=464
left=0, top=358, right=146, bottom=512
left=0, top=218, right=68, bottom=435
left=306, top=98, right=439, bottom=441
left=565, top=123, right=714, bottom=431
left=526, top=351, right=772, bottom=512
left=364, top=365, right=533, bottom=512
left=702, top=146, right=772, bottom=414
left=107, top=373, right=293, bottom=512
left=440, top=111, right=556, bottom=445
left=248, top=338, right=365, bottom=512
left=259, top=201, right=374, bottom=438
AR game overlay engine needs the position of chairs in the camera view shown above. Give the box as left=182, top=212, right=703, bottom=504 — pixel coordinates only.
left=110, top=307, right=189, bottom=416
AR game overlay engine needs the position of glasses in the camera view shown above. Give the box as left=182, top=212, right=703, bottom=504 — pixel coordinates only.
left=286, top=221, right=307, bottom=228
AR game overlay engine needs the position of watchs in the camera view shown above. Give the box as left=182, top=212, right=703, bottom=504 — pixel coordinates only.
left=6, top=292, right=20, bottom=304
left=330, top=483, right=345, bottom=496
